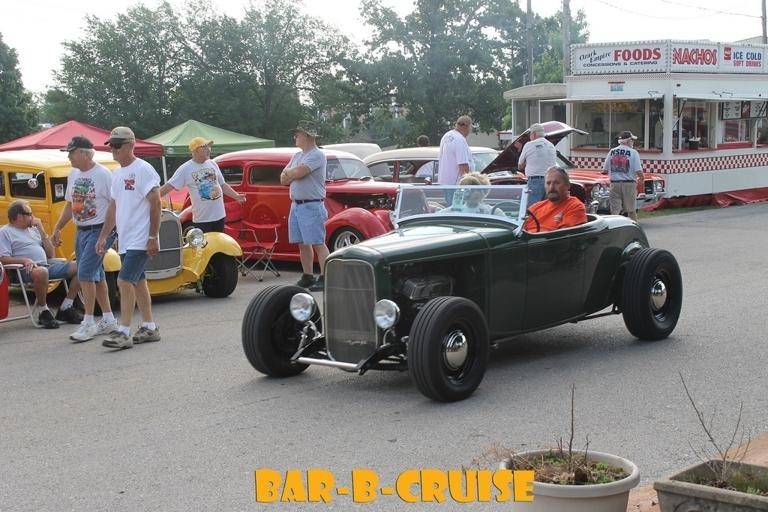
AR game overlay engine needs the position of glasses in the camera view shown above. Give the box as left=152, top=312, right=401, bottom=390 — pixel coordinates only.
left=109, top=142, right=127, bottom=150
left=21, top=212, right=32, bottom=217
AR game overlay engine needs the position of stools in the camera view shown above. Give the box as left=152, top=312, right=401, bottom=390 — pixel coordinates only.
left=0, top=263, right=72, bottom=329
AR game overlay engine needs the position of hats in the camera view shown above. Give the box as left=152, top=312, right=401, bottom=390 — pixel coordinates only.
left=189, top=137, right=214, bottom=152
left=288, top=120, right=320, bottom=137
left=104, top=127, right=135, bottom=145
left=619, top=131, right=638, bottom=140
left=60, top=136, right=93, bottom=151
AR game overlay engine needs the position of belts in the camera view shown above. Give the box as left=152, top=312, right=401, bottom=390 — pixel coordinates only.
left=295, top=198, right=323, bottom=205
left=531, top=176, right=544, bottom=179
left=611, top=180, right=636, bottom=183
left=78, top=224, right=103, bottom=230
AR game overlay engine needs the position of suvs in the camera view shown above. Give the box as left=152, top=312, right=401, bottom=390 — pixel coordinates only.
left=241, top=184, right=682, bottom=402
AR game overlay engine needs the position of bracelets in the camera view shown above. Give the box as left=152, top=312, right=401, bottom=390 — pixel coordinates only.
left=40, top=233, right=48, bottom=240
left=149, top=236, right=160, bottom=241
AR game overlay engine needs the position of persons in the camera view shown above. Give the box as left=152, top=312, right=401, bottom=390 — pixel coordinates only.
left=521, top=165, right=588, bottom=233
left=159, top=136, right=247, bottom=231
left=434, top=171, right=510, bottom=219
left=50, top=135, right=118, bottom=341
left=278, top=120, right=331, bottom=291
left=0, top=199, right=83, bottom=327
left=437, top=116, right=475, bottom=206
left=517, top=124, right=557, bottom=212
left=94, top=126, right=163, bottom=351
left=603, top=130, right=647, bottom=223
left=415, top=135, right=429, bottom=146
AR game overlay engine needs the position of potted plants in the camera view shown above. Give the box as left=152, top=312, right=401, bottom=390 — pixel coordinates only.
left=653, top=373, right=767, bottom=511
left=500, top=385, right=639, bottom=510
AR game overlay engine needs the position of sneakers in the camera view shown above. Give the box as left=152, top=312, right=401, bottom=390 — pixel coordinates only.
left=38, top=307, right=161, bottom=349
left=297, top=274, right=316, bottom=288
left=309, top=275, right=324, bottom=291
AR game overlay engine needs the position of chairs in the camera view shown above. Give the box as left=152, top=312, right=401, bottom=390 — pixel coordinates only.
left=223, top=201, right=282, bottom=283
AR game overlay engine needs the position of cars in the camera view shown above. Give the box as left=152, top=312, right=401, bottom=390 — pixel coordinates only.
left=495, top=148, right=667, bottom=218
left=0, top=149, right=243, bottom=316
left=159, top=147, right=446, bottom=264
left=318, top=121, right=602, bottom=214
